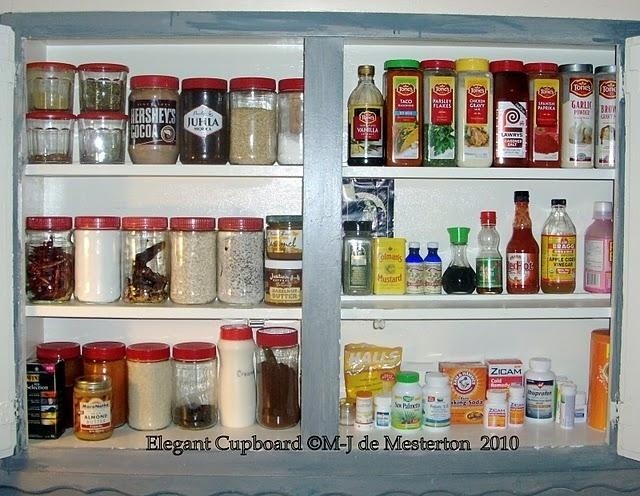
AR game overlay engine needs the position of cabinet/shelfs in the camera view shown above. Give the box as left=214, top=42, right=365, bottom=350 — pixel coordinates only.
left=24, top=161, right=304, bottom=452
left=344, top=165, right=617, bottom=450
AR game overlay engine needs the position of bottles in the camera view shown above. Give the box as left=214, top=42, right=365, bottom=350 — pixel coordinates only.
left=23, top=60, right=304, bottom=166
left=483, top=356, right=589, bottom=432
left=24, top=215, right=265, bottom=306
left=404, top=190, right=613, bottom=295
left=347, top=58, right=617, bottom=169
left=354, top=371, right=454, bottom=434
left=34, top=320, right=301, bottom=430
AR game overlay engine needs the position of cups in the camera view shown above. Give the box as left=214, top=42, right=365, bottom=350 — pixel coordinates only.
left=337, top=397, right=357, bottom=426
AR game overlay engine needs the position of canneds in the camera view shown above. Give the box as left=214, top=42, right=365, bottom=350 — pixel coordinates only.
left=373, top=238, right=407, bottom=295
left=128, top=73, right=306, bottom=164
left=20, top=211, right=301, bottom=309
left=383, top=58, right=618, bottom=169
left=36, top=327, right=302, bottom=442
